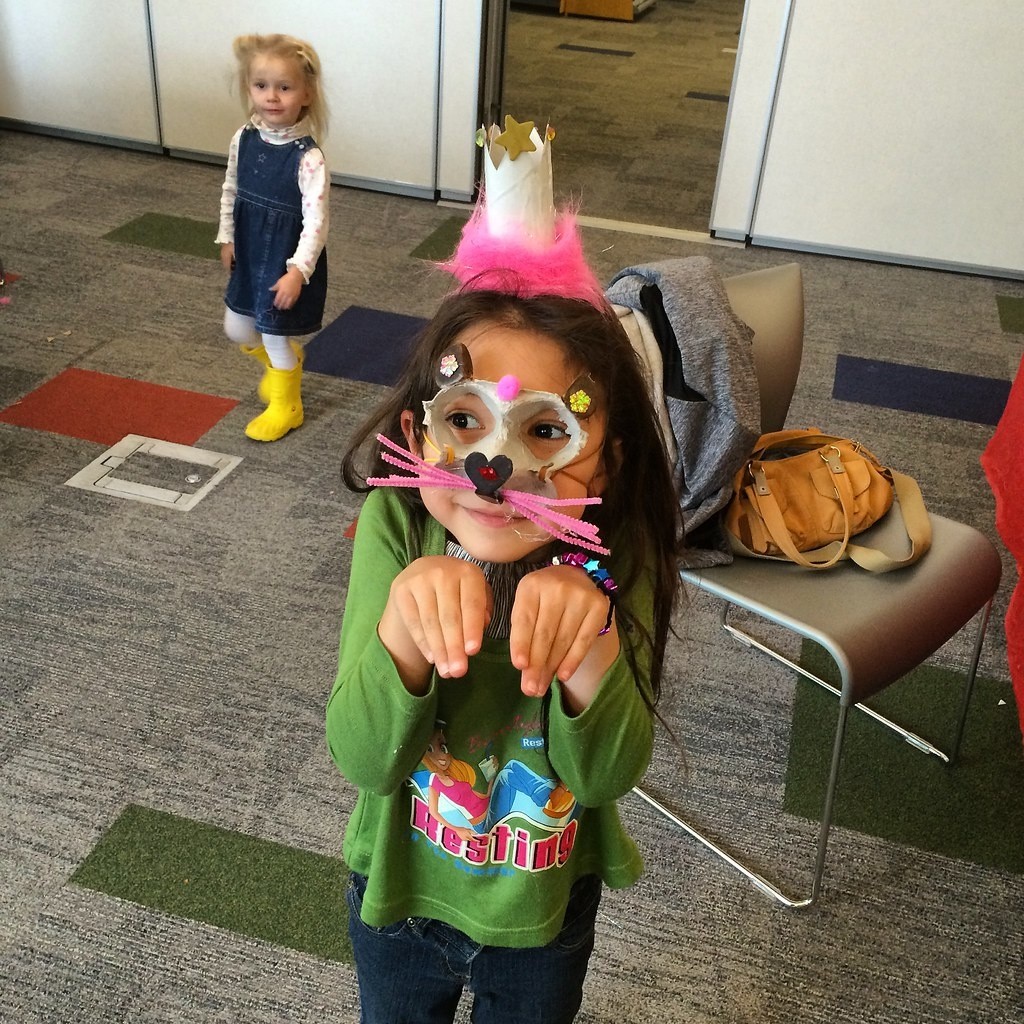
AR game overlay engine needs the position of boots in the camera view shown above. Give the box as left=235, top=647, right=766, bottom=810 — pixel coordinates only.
left=245, top=357, right=304, bottom=443
left=238, top=340, right=305, bottom=406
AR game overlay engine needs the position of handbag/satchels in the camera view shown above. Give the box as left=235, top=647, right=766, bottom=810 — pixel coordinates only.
left=720, top=428, right=934, bottom=572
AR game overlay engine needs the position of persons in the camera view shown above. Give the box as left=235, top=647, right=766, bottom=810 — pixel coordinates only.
left=325, top=290, right=691, bottom=1024
left=214, top=35, right=329, bottom=442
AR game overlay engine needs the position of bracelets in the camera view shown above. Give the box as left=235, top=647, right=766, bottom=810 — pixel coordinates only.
left=545, top=552, right=619, bottom=636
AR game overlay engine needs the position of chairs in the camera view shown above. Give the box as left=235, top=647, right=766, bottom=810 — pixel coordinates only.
left=608, top=261, right=1002, bottom=910
